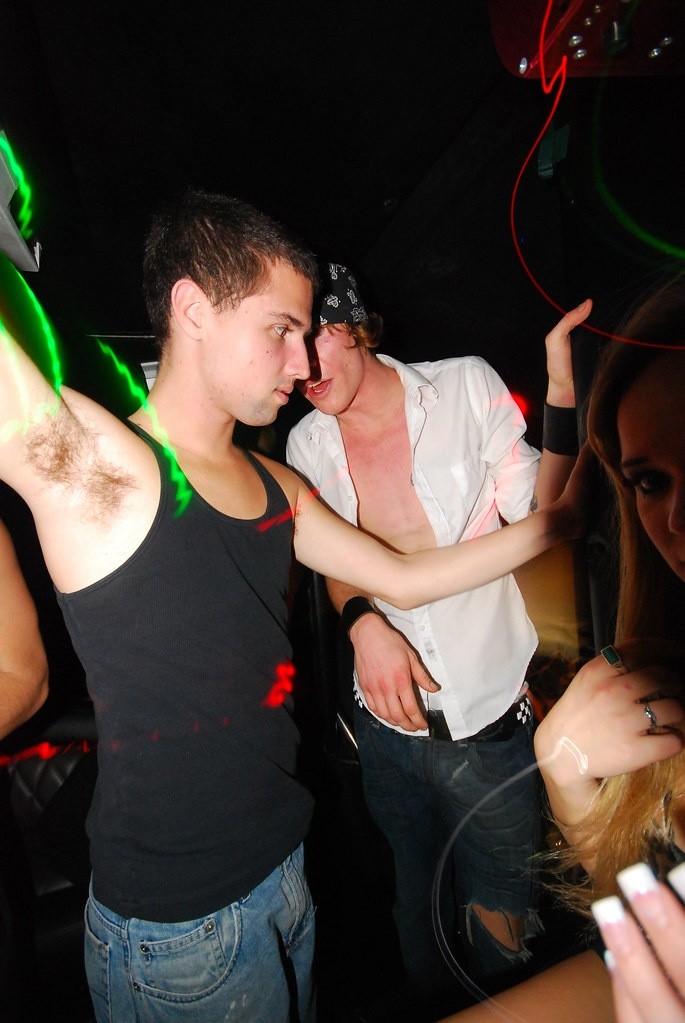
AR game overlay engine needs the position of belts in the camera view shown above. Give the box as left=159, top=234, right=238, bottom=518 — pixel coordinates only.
left=351, top=683, right=534, bottom=743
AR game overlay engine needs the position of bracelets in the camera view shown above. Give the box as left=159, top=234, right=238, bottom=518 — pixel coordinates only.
left=341, top=596, right=379, bottom=639
left=541, top=402, right=579, bottom=457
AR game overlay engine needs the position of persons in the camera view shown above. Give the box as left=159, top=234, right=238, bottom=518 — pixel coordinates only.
left=0, top=190, right=685, bottom=1023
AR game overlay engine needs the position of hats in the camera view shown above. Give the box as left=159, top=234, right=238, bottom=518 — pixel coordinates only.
left=308, top=260, right=370, bottom=326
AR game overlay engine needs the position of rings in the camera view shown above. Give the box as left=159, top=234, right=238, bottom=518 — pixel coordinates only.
left=645, top=702, right=656, bottom=727
left=601, top=645, right=628, bottom=673
